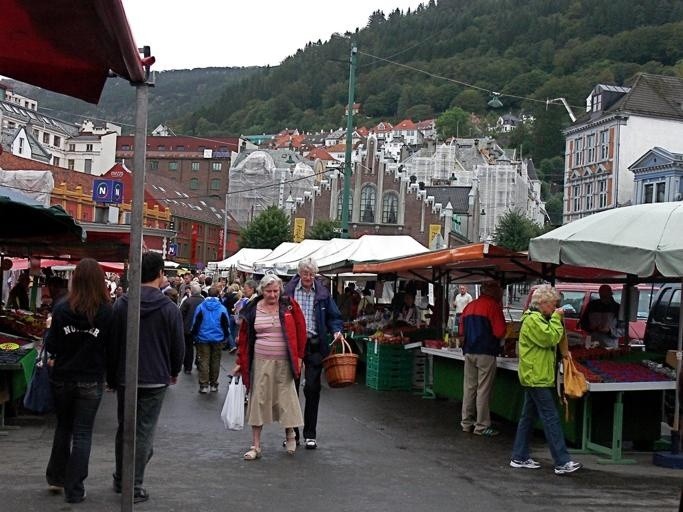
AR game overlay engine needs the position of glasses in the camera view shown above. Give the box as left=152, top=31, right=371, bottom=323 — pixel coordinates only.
left=302, top=272, right=315, bottom=279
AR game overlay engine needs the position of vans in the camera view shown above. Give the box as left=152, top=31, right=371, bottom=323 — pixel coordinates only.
left=645, top=282, right=682, bottom=353
left=524, top=283, right=659, bottom=346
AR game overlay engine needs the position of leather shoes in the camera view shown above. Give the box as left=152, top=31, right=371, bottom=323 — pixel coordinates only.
left=113, top=478, right=122, bottom=493
left=133, top=486, right=148, bottom=503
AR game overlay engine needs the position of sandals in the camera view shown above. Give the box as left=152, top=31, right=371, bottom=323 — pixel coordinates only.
left=285, top=431, right=296, bottom=455
left=303, top=437, right=316, bottom=449
left=243, top=445, right=261, bottom=460
left=282, top=438, right=299, bottom=446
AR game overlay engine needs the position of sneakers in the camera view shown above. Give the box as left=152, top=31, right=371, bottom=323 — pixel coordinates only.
left=209, top=384, right=217, bottom=392
left=198, top=385, right=208, bottom=393
left=46, top=484, right=64, bottom=495
left=462, top=425, right=472, bottom=432
left=472, top=427, right=500, bottom=436
left=64, top=488, right=86, bottom=503
left=553, top=460, right=581, bottom=475
left=508, top=457, right=542, bottom=469
left=228, top=346, right=237, bottom=353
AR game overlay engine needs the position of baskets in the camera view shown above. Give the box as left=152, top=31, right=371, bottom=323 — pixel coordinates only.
left=321, top=337, right=359, bottom=388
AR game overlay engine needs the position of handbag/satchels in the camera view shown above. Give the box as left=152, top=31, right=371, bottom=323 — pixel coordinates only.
left=23, top=336, right=55, bottom=413
left=365, top=303, right=374, bottom=315
left=562, top=354, right=587, bottom=400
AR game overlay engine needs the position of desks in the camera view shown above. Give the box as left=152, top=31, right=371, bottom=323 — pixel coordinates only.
left=0, top=332, right=51, bottom=435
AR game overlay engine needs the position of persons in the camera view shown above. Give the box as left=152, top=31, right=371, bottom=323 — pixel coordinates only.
left=505, top=285, right=585, bottom=477
left=282, top=255, right=342, bottom=451
left=226, top=273, right=307, bottom=462
left=111, top=251, right=187, bottom=502
left=1, top=254, right=472, bottom=378
left=579, top=284, right=625, bottom=349
left=188, top=286, right=235, bottom=394
left=457, top=280, right=507, bottom=438
left=40, top=256, right=117, bottom=504
